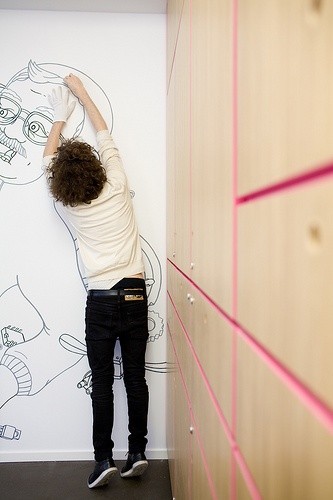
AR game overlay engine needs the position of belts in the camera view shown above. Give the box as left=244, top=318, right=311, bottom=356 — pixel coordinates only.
left=87, top=290, right=146, bottom=297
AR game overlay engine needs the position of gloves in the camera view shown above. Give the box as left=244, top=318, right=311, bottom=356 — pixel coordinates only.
left=48, top=86, right=76, bottom=123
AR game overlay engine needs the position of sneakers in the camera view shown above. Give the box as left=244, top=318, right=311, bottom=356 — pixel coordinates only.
left=120, top=451, right=149, bottom=478
left=87, top=457, right=118, bottom=489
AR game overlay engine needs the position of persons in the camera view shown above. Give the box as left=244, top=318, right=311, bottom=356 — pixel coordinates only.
left=42, top=74, right=149, bottom=489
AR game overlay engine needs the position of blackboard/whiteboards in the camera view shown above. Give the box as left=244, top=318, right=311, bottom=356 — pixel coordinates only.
left=0, top=9, right=168, bottom=462
